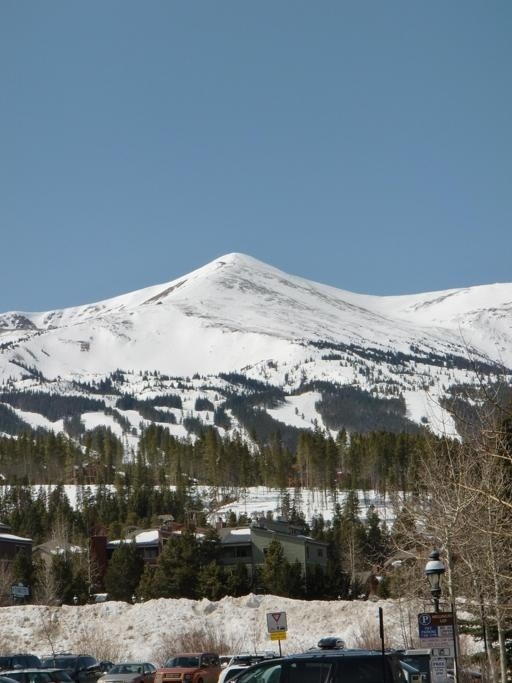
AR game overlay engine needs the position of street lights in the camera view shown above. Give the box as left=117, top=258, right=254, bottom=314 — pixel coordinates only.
left=424, top=543, right=446, bottom=612
